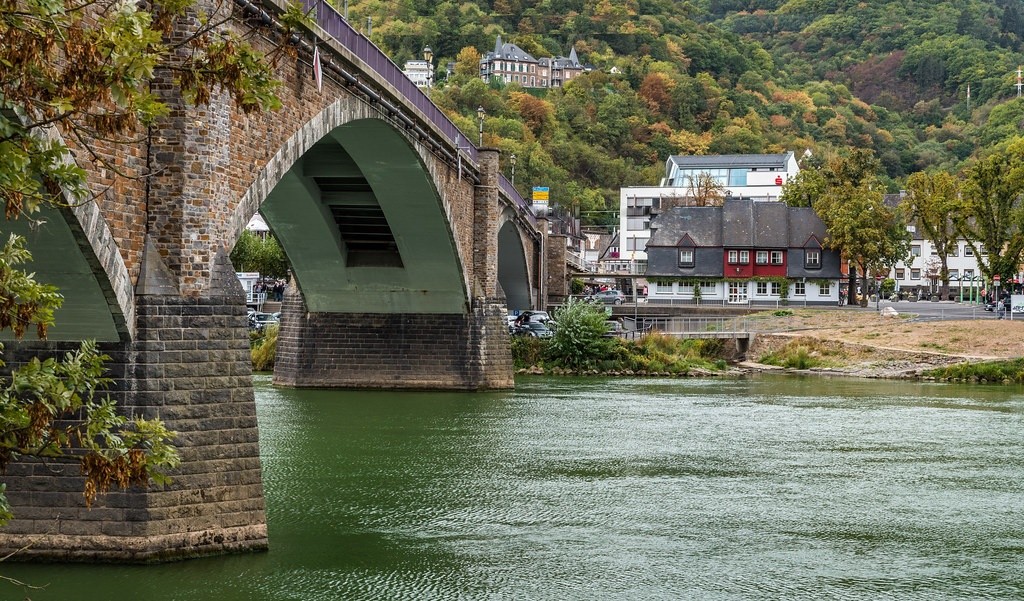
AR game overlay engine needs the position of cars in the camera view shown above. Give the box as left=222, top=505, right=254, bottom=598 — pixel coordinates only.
left=984, top=297, right=1012, bottom=313
left=507, top=310, right=555, bottom=340
left=247, top=307, right=281, bottom=330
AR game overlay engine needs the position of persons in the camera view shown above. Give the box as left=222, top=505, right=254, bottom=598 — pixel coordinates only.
left=643, top=285, right=648, bottom=302
left=838, top=283, right=930, bottom=302
left=580, top=283, right=624, bottom=303
left=253, top=275, right=288, bottom=301
left=514, top=315, right=525, bottom=336
left=980, top=286, right=1024, bottom=320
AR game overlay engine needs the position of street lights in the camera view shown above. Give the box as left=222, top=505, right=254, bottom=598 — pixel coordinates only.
left=476, top=105, right=486, bottom=147
left=423, top=44, right=434, bottom=97
left=510, top=154, right=516, bottom=185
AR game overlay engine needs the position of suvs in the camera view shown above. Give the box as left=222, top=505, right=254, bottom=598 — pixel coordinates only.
left=584, top=290, right=626, bottom=305
